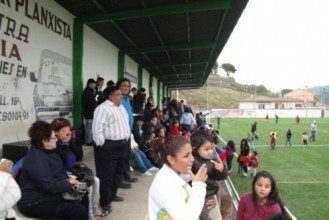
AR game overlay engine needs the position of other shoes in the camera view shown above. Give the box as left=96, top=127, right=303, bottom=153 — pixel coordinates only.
left=148, top=166, right=159, bottom=171
left=141, top=171, right=153, bottom=176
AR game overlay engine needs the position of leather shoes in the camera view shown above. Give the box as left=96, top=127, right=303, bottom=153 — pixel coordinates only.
left=128, top=178, right=137, bottom=182
left=119, top=183, right=131, bottom=188
left=112, top=196, right=123, bottom=201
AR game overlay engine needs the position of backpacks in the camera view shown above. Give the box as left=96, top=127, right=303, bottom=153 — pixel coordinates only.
left=62, top=172, right=87, bottom=201
left=71, top=163, right=94, bottom=186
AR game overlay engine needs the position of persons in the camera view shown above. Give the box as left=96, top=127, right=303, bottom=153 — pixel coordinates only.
left=148, top=130, right=236, bottom=220
left=296, top=116, right=300, bottom=123
left=217, top=117, right=220, bottom=129
left=82, top=76, right=115, bottom=146
left=302, top=132, right=309, bottom=146
left=92, top=86, right=129, bottom=217
left=265, top=114, right=268, bottom=122
left=47, top=118, right=106, bottom=220
left=285, top=129, right=292, bottom=146
left=0, top=158, right=22, bottom=220
left=17, top=120, right=90, bottom=220
left=236, top=171, right=289, bottom=220
left=237, top=121, right=259, bottom=178
left=129, top=87, right=212, bottom=176
left=275, top=114, right=278, bottom=123
left=116, top=78, right=138, bottom=189
left=309, top=121, right=317, bottom=140
left=270, top=128, right=277, bottom=149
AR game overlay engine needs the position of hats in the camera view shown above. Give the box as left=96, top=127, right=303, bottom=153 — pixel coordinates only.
left=88, top=79, right=97, bottom=83
